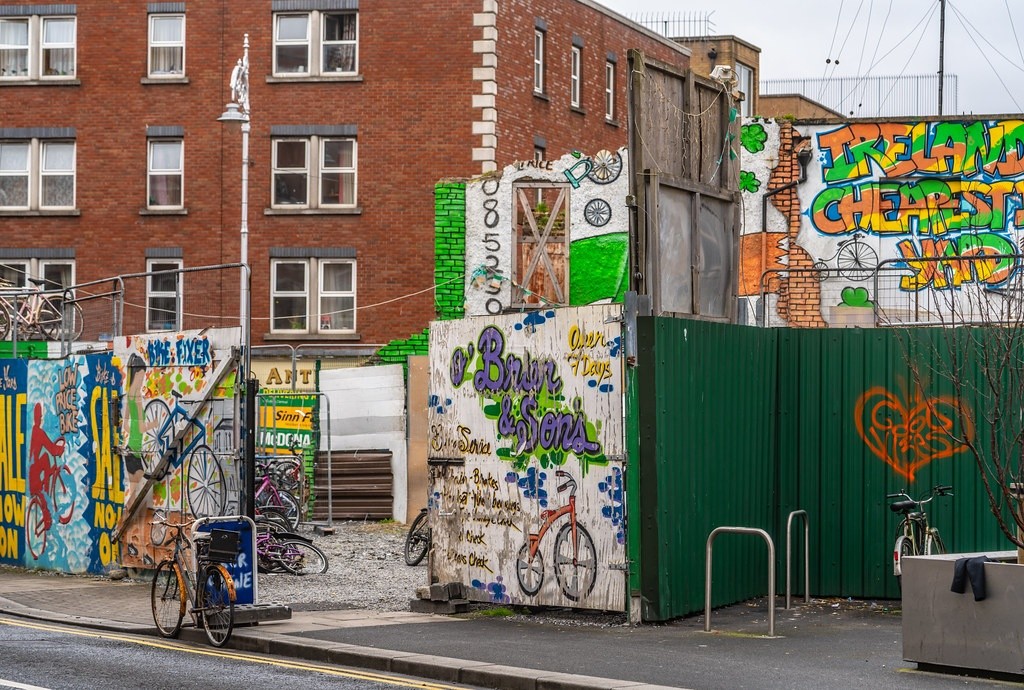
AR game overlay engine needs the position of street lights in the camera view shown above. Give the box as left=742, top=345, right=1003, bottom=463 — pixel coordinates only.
left=217, top=32, right=252, bottom=358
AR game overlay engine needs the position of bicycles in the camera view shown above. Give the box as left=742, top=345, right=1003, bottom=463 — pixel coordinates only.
left=253, top=460, right=328, bottom=576
left=0, top=278, right=83, bottom=342
left=148, top=520, right=234, bottom=648
left=404, top=508, right=428, bottom=565
left=886, top=486, right=943, bottom=596
